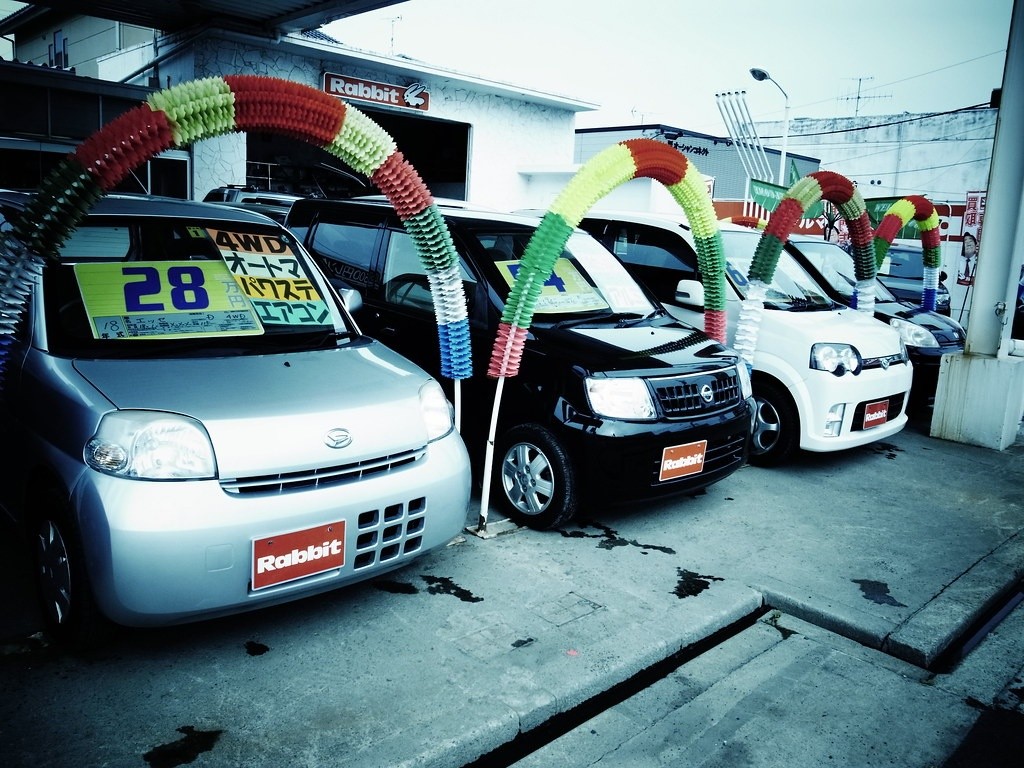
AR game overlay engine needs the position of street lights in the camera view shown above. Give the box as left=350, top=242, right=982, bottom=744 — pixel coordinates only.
left=749, top=66, right=790, bottom=186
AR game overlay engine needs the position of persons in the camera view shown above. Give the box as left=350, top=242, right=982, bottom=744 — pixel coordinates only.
left=958, top=232, right=978, bottom=282
left=824, top=224, right=839, bottom=246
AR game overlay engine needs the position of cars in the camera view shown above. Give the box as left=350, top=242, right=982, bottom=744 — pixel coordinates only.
left=0, top=189, right=474, bottom=652
left=280, top=199, right=756, bottom=533
left=782, top=238, right=966, bottom=426
left=201, top=181, right=322, bottom=229
left=565, top=203, right=916, bottom=465
left=843, top=242, right=953, bottom=318
